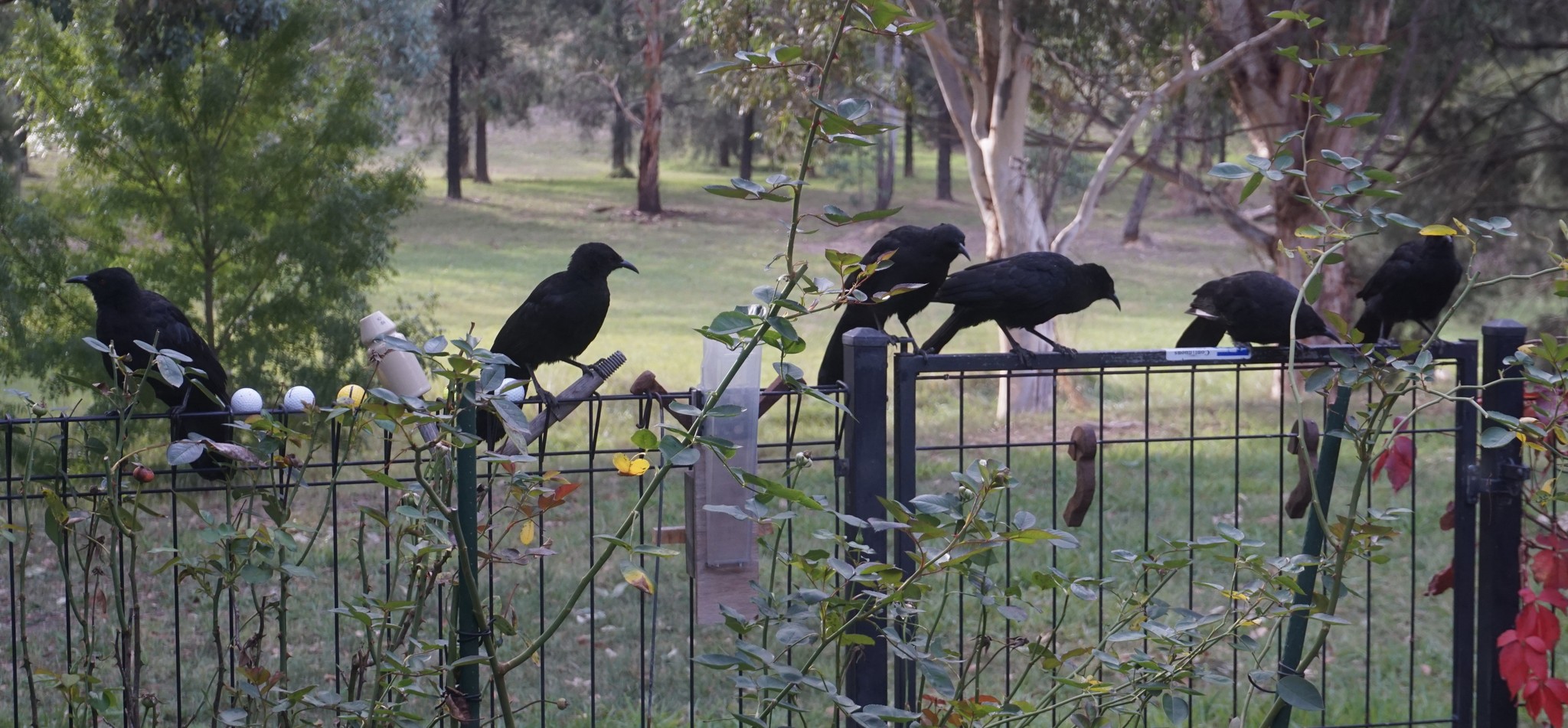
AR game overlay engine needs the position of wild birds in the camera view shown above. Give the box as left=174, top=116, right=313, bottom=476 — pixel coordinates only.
left=474, top=242, right=639, bottom=443
left=819, top=222, right=1465, bottom=394
left=66, top=266, right=239, bottom=482
